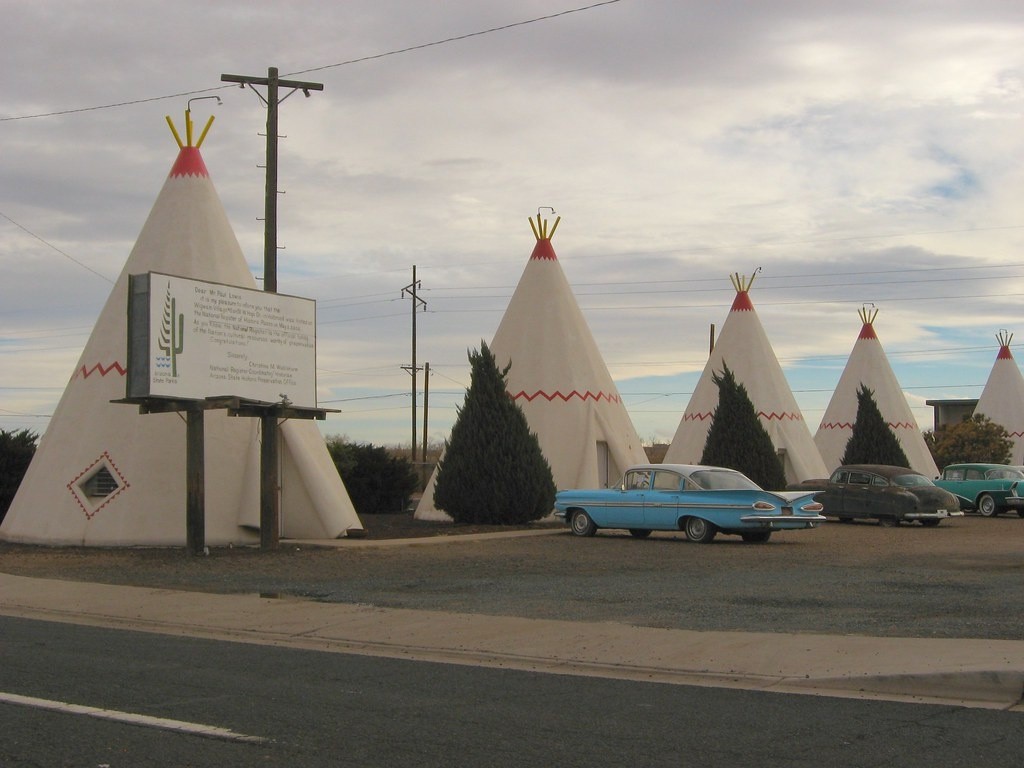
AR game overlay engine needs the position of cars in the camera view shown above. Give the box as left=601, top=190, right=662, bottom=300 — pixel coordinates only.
left=908, top=463, right=1024, bottom=519
left=784, top=462, right=964, bottom=528
left=552, top=462, right=826, bottom=546
